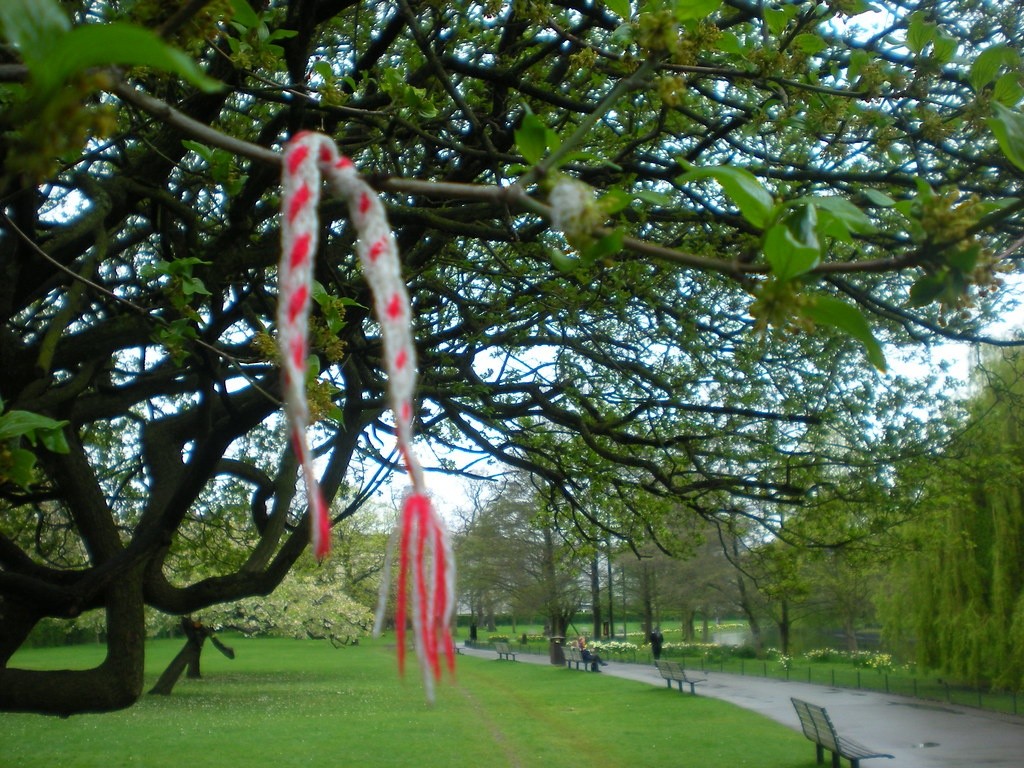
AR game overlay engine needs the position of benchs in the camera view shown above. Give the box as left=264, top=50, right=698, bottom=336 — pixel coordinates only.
left=791, top=697, right=896, bottom=768
left=655, top=660, right=708, bottom=695
left=560, top=646, right=597, bottom=672
left=493, top=642, right=518, bottom=661
left=452, top=637, right=466, bottom=655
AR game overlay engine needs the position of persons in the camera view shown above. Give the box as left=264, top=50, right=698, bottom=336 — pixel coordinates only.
left=572, top=636, right=607, bottom=672
left=650, top=627, right=665, bottom=667
